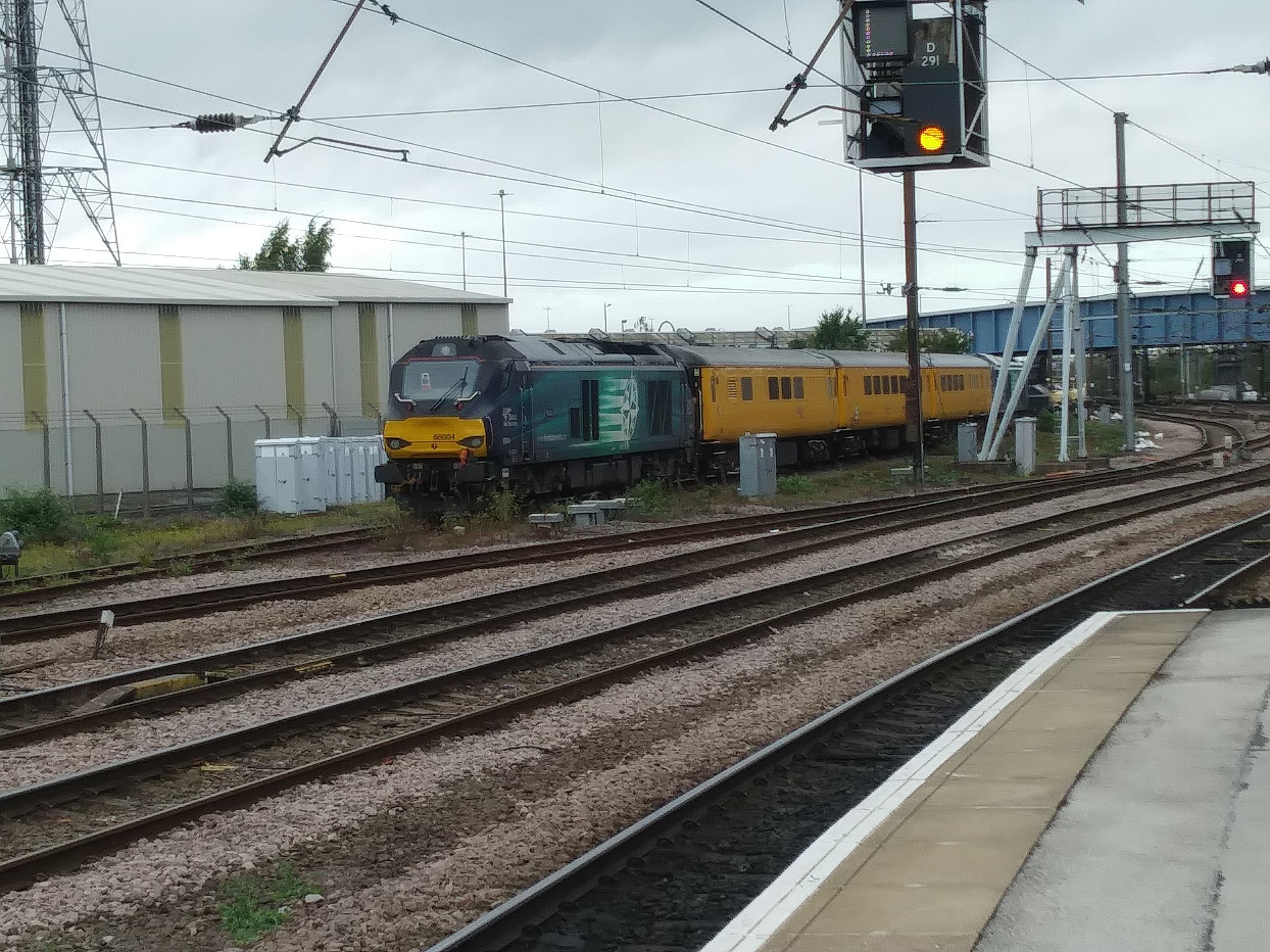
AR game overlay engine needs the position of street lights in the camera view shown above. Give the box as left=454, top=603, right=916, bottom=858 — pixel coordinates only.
left=785, top=305, right=794, bottom=329
left=603, top=301, right=611, bottom=332
left=491, top=190, right=514, bottom=294
left=543, top=308, right=553, bottom=329
left=455, top=232, right=474, bottom=291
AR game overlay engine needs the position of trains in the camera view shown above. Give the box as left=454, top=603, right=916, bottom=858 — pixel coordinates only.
left=374, top=333, right=1030, bottom=520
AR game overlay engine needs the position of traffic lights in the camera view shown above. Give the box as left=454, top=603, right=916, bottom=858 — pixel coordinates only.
left=851, top=0, right=913, bottom=63
left=1213, top=257, right=1233, bottom=275
left=900, top=64, right=962, bottom=156
left=1228, top=258, right=1249, bottom=298
